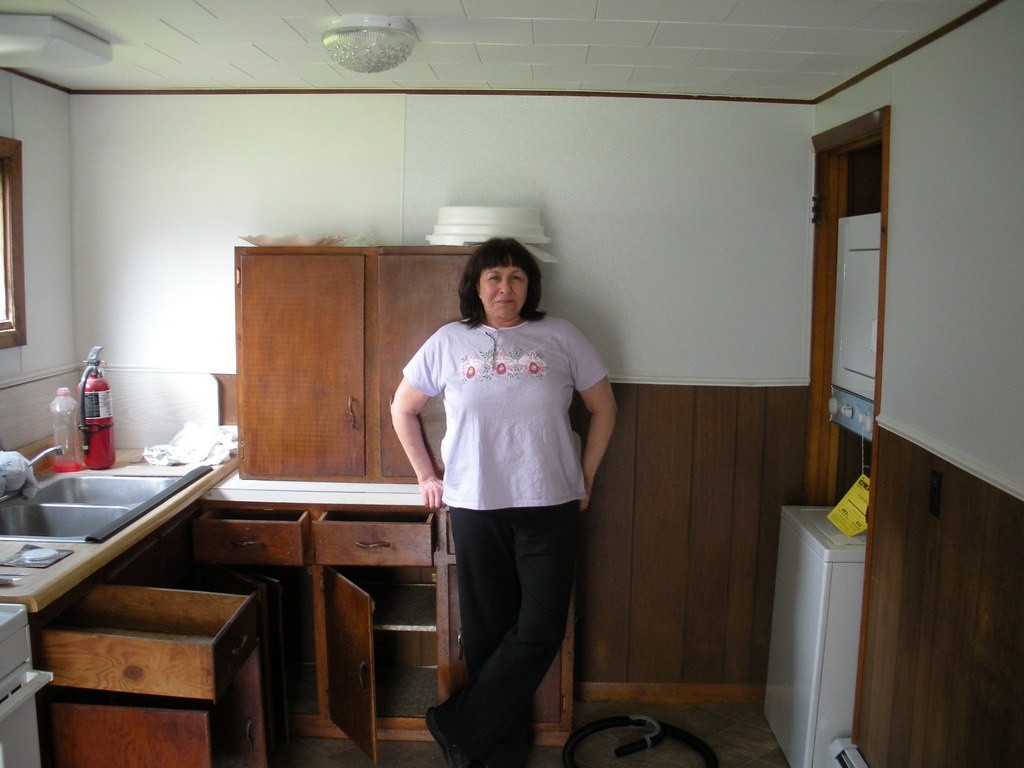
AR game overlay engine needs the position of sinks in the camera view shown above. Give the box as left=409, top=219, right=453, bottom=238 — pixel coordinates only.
left=0, top=505, right=152, bottom=542
left=35, top=475, right=177, bottom=506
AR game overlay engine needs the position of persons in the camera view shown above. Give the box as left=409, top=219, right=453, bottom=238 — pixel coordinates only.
left=390, top=237, right=618, bottom=768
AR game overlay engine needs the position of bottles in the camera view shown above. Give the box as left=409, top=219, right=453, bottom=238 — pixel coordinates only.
left=48, top=387, right=83, bottom=473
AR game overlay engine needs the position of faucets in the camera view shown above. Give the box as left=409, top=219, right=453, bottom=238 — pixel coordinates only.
left=28, top=445, right=64, bottom=467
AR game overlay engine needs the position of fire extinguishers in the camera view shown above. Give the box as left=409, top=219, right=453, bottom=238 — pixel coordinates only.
left=77, top=346, right=116, bottom=469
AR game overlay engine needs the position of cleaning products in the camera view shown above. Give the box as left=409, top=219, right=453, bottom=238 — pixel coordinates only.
left=50, top=388, right=83, bottom=473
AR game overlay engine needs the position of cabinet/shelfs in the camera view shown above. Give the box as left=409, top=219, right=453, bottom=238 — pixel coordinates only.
left=37, top=511, right=577, bottom=768
left=234, top=244, right=482, bottom=485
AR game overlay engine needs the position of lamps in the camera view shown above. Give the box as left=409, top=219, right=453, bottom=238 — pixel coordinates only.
left=0, top=14, right=113, bottom=70
left=323, top=15, right=420, bottom=72
